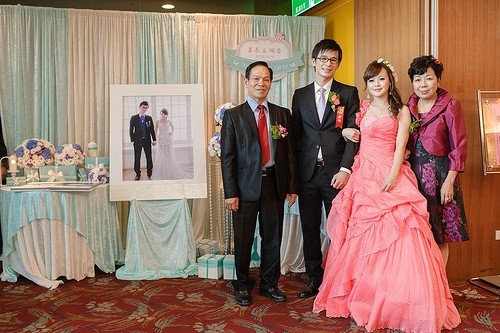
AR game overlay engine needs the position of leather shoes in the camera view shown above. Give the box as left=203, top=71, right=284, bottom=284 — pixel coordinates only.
left=298, top=281, right=320, bottom=298
left=260, top=286, right=286, bottom=302
left=235, top=291, right=252, bottom=305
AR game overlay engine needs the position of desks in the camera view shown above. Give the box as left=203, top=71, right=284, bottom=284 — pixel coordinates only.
left=115, top=198, right=199, bottom=279
left=0, top=181, right=126, bottom=292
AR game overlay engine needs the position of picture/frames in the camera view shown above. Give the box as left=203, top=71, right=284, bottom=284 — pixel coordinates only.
left=108, top=84, right=207, bottom=203
left=477, top=90, right=500, bottom=176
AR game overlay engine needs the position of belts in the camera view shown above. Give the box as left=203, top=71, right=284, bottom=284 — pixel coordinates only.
left=316, top=160, right=325, bottom=166
left=262, top=170, right=276, bottom=177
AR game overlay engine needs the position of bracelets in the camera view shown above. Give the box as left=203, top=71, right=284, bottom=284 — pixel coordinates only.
left=389, top=175, right=397, bottom=182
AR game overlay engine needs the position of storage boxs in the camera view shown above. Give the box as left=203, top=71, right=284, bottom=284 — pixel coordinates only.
left=6, top=157, right=109, bottom=185
left=195, top=238, right=238, bottom=281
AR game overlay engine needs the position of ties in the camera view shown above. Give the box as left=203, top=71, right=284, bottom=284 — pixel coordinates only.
left=317, top=88, right=326, bottom=158
left=257, top=105, right=270, bottom=166
left=141, top=115, right=146, bottom=139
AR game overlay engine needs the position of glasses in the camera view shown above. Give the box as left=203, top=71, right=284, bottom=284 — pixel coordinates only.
left=316, top=57, right=339, bottom=64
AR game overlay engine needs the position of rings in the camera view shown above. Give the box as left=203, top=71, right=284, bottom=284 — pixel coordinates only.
left=445, top=197, right=449, bottom=201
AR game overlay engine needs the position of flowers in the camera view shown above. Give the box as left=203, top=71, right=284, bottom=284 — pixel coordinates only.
left=212, top=102, right=238, bottom=127
left=207, top=132, right=223, bottom=160
left=11, top=136, right=56, bottom=169
left=85, top=164, right=111, bottom=184
left=354, top=100, right=372, bottom=128
left=271, top=121, right=289, bottom=141
left=53, top=144, right=87, bottom=167
left=328, top=91, right=341, bottom=113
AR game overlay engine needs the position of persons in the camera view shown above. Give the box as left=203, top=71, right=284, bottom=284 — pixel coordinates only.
left=291, top=39, right=360, bottom=298
left=404, top=55, right=470, bottom=268
left=152, top=109, right=178, bottom=180
left=129, top=101, right=156, bottom=181
left=343, top=58, right=411, bottom=325
left=220, top=62, right=298, bottom=306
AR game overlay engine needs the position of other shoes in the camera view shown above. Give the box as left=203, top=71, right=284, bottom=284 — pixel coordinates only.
left=147, top=174, right=152, bottom=180
left=135, top=174, right=140, bottom=180
